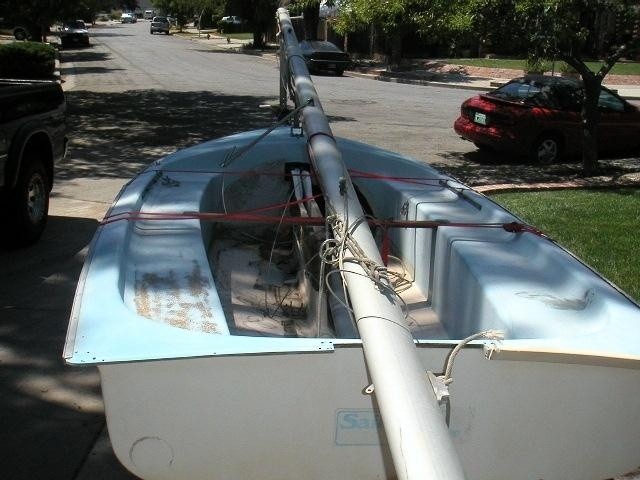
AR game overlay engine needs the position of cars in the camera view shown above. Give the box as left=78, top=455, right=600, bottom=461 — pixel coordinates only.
left=0, top=8, right=174, bottom=47
left=296, top=36, right=353, bottom=77
left=221, top=15, right=241, bottom=23
left=453, top=73, right=640, bottom=164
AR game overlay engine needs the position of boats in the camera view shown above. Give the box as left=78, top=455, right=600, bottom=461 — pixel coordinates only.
left=58, top=114, right=640, bottom=480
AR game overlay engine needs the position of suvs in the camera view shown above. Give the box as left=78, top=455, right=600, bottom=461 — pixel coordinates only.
left=0, top=73, right=75, bottom=250
